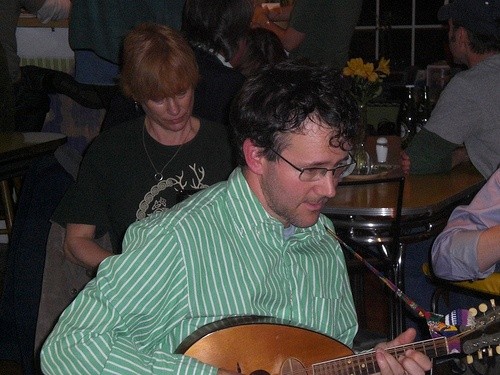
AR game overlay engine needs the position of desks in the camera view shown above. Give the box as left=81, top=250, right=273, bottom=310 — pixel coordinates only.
left=0, top=132, right=67, bottom=242
left=16, top=27, right=108, bottom=181
left=321, top=134, right=487, bottom=333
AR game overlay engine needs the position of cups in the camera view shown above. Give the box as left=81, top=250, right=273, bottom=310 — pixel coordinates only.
left=376, top=136, right=388, bottom=162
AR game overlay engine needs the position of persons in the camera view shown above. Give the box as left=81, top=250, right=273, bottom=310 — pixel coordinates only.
left=430, top=162, right=500, bottom=281
left=250, top=0, right=361, bottom=68
left=405, top=0, right=500, bottom=175
left=1, top=0, right=24, bottom=109
left=39, top=60, right=433, bottom=375
left=67, top=0, right=186, bottom=84
left=51, top=21, right=235, bottom=272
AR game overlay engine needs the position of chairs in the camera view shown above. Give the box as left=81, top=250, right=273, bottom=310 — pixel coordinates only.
left=321, top=177, right=406, bottom=343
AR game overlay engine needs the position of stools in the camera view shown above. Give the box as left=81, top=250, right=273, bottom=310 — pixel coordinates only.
left=423, top=261, right=500, bottom=315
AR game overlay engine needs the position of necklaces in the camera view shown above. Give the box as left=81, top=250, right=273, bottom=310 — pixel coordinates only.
left=142, top=109, right=192, bottom=181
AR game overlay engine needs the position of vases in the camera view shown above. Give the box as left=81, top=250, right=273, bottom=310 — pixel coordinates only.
left=350, top=107, right=372, bottom=175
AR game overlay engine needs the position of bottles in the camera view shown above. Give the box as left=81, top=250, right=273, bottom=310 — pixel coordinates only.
left=397, top=86, right=417, bottom=148
left=417, top=85, right=432, bottom=129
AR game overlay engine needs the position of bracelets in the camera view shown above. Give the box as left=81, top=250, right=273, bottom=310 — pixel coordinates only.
left=263, top=19, right=270, bottom=26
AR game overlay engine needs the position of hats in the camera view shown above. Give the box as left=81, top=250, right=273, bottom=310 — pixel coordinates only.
left=437, top=0, right=500, bottom=22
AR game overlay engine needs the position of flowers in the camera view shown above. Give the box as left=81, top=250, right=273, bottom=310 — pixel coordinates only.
left=343, top=57, right=390, bottom=109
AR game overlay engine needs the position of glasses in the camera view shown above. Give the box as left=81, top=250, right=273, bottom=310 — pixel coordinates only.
left=253, top=136, right=357, bottom=183
